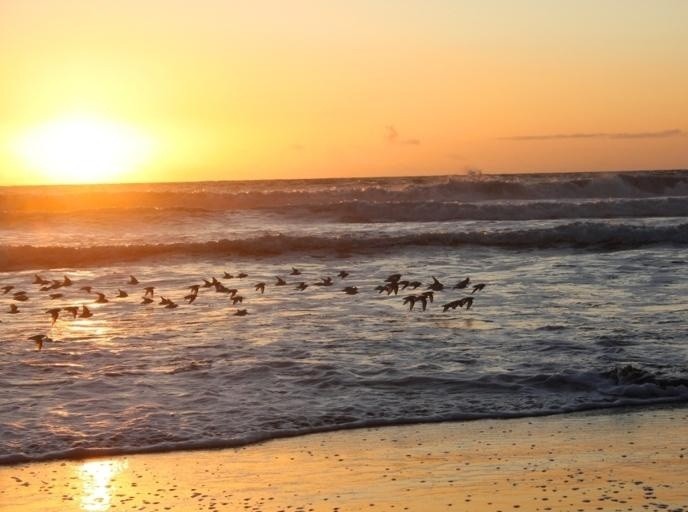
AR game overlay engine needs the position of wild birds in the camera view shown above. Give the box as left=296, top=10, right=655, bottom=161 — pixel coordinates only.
left=1, top=272, right=250, bottom=327
left=376, top=273, right=487, bottom=312
left=27, top=333, right=65, bottom=350
left=255, top=268, right=362, bottom=296
